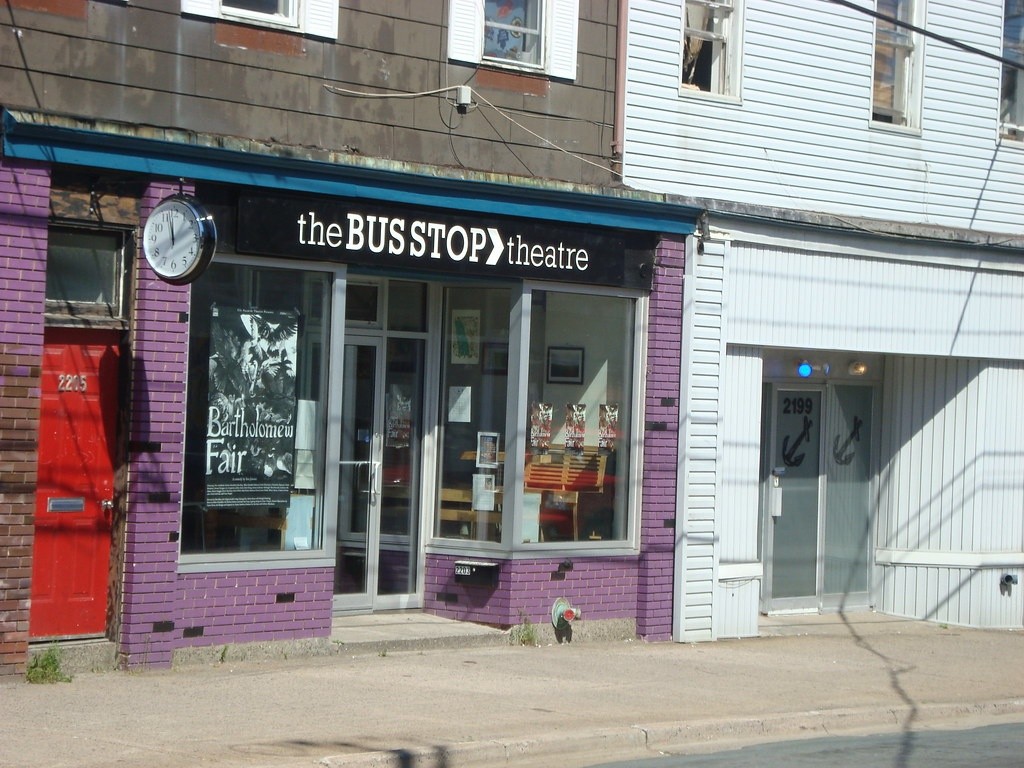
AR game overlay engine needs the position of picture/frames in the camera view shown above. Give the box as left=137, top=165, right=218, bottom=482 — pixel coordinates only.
left=547, top=345, right=585, bottom=386
left=480, top=340, right=510, bottom=376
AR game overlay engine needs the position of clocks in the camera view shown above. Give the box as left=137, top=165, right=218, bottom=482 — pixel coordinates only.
left=140, top=194, right=218, bottom=285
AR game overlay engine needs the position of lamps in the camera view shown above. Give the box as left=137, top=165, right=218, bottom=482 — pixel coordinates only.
left=793, top=358, right=831, bottom=379
